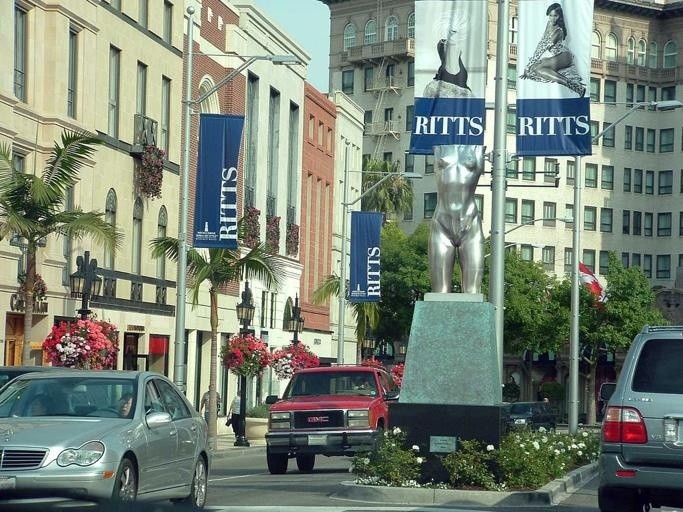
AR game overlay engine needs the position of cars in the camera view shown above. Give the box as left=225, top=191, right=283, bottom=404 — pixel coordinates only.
left=0, top=367, right=212, bottom=512
left=0, top=366, right=112, bottom=418
left=507, top=400, right=557, bottom=434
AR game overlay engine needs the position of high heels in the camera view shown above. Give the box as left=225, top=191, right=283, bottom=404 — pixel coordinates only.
left=568, top=79, right=588, bottom=98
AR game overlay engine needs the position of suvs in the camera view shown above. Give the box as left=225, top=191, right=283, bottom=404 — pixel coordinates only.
left=595, top=323, right=683, bottom=512
left=262, top=362, right=401, bottom=477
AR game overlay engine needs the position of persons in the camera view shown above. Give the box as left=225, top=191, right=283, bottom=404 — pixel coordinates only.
left=30, top=393, right=55, bottom=416
left=519, top=3, right=585, bottom=98
left=200, top=384, right=220, bottom=425
left=428, top=143, right=485, bottom=295
left=227, top=391, right=241, bottom=438
left=118, top=392, right=133, bottom=417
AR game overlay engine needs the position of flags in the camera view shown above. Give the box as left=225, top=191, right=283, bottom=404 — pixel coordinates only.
left=578, top=260, right=609, bottom=308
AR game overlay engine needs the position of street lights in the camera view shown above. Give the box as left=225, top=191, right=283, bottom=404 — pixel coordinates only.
left=334, top=146, right=423, bottom=366
left=68, top=250, right=102, bottom=323
left=172, top=3, right=304, bottom=402
left=563, top=97, right=682, bottom=436
left=285, top=292, right=304, bottom=349
left=233, top=280, right=256, bottom=449
